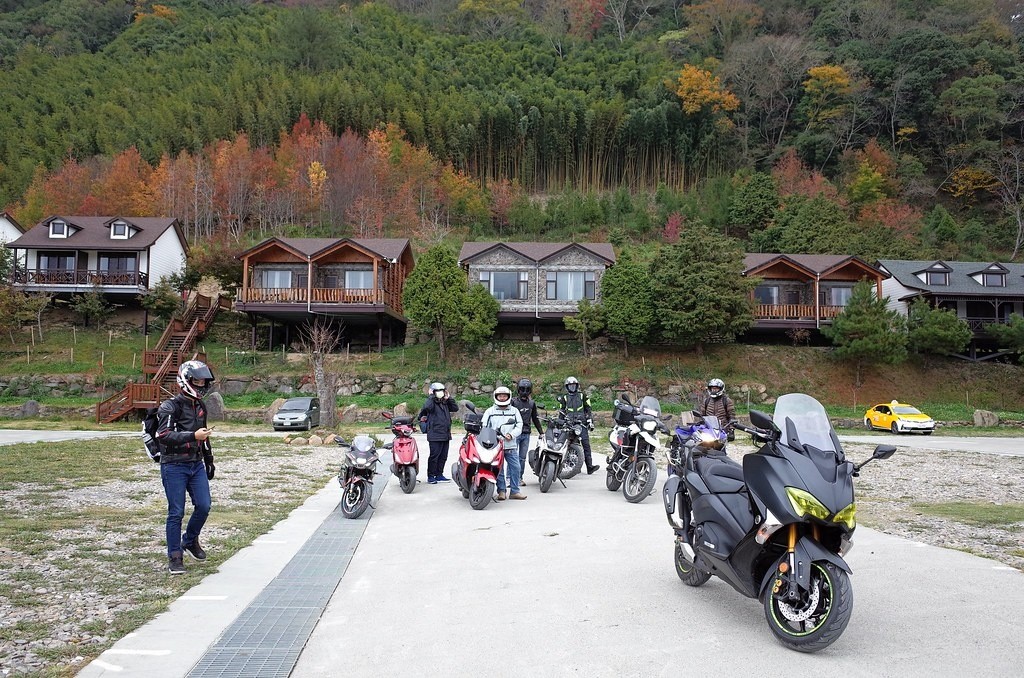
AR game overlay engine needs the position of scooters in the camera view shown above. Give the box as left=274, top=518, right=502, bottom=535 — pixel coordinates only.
left=333, top=434, right=383, bottom=520
left=665, top=409, right=739, bottom=479
left=605, top=392, right=673, bottom=504
left=660, top=392, right=898, bottom=654
left=450, top=401, right=516, bottom=511
left=381, top=411, right=428, bottom=494
left=528, top=403, right=592, bottom=494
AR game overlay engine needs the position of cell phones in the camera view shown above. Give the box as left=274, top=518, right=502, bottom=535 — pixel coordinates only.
left=206, top=425, right=215, bottom=431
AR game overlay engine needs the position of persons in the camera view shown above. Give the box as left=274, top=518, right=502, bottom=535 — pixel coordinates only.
left=558, top=376, right=600, bottom=474
left=699, top=378, right=737, bottom=454
left=155, top=360, right=215, bottom=574
left=506, top=379, right=545, bottom=487
left=481, top=386, right=527, bottom=499
left=418, top=383, right=459, bottom=484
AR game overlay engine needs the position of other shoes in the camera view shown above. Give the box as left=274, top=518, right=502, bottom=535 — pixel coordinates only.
left=519, top=477, right=526, bottom=486
left=508, top=491, right=527, bottom=499
left=497, top=491, right=506, bottom=500
left=505, top=479, right=511, bottom=488
left=587, top=465, right=600, bottom=475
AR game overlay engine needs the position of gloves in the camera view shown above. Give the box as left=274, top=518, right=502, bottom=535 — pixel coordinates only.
left=205, top=454, right=216, bottom=478
left=589, top=422, right=594, bottom=431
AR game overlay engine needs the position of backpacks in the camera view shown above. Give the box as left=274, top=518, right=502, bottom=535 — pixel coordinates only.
left=420, top=396, right=434, bottom=434
left=141, top=397, right=206, bottom=462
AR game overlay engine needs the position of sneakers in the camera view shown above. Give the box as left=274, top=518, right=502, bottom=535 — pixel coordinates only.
left=181, top=533, right=206, bottom=561
left=434, top=474, right=451, bottom=482
left=169, top=551, right=186, bottom=574
left=428, top=476, right=437, bottom=484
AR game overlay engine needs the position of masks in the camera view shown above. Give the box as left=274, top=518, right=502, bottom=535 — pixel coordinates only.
left=568, top=386, right=576, bottom=392
left=435, top=391, right=445, bottom=398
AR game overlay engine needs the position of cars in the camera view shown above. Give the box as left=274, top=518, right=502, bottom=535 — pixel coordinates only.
left=270, top=396, right=321, bottom=432
left=863, top=400, right=937, bottom=436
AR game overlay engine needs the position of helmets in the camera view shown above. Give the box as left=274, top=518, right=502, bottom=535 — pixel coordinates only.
left=176, top=361, right=211, bottom=399
left=493, top=387, right=512, bottom=406
left=428, top=382, right=446, bottom=401
left=707, top=378, right=725, bottom=397
left=565, top=377, right=580, bottom=395
left=517, top=378, right=532, bottom=397
